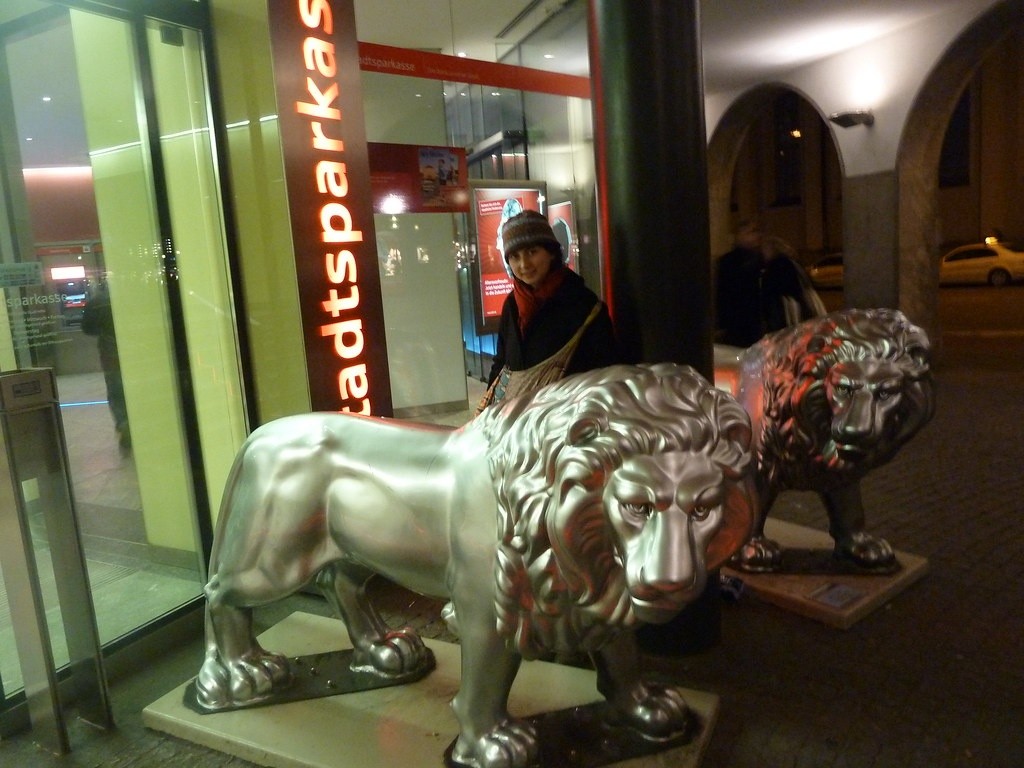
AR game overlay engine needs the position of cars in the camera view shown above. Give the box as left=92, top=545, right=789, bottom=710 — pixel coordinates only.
left=802, top=251, right=843, bottom=290
left=940, top=236, right=1024, bottom=288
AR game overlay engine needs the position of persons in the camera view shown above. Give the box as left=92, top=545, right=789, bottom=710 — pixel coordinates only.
left=710, top=220, right=805, bottom=350
left=80, top=281, right=131, bottom=449
left=486, top=208, right=631, bottom=379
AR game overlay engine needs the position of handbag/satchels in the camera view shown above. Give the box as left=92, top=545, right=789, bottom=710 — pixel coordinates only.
left=474, top=303, right=605, bottom=421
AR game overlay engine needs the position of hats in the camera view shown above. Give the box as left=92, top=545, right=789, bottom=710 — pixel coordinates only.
left=502, top=209, right=562, bottom=264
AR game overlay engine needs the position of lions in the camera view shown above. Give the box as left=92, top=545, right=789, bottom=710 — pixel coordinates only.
left=194, top=362, right=754, bottom=768
left=714, top=308, right=935, bottom=574
